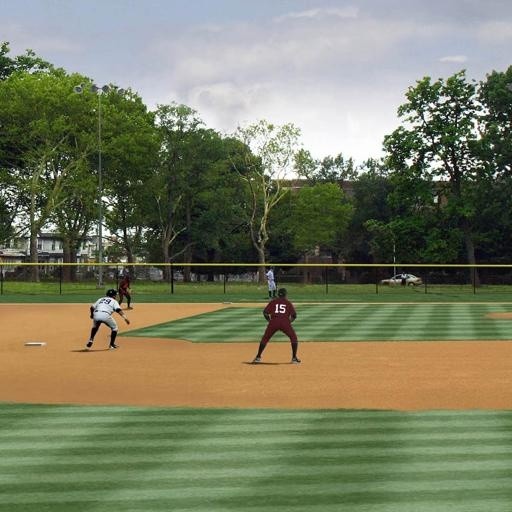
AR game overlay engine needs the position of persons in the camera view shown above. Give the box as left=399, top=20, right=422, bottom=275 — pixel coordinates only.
left=400, top=271, right=407, bottom=288
left=84, top=289, right=130, bottom=349
left=251, top=288, right=302, bottom=364
left=264, top=266, right=277, bottom=299
left=117, top=274, right=133, bottom=310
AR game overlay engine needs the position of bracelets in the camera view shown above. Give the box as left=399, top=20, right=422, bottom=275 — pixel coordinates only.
left=121, top=314, right=128, bottom=321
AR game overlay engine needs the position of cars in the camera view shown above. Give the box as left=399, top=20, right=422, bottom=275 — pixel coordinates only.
left=3, top=266, right=184, bottom=282
left=380, top=273, right=424, bottom=287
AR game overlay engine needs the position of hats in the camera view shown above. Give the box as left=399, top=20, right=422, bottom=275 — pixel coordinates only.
left=278, top=287, right=288, bottom=296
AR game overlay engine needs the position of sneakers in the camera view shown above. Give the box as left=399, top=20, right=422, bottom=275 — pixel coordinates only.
left=86, top=339, right=93, bottom=347
left=290, top=357, right=302, bottom=363
left=253, top=356, right=261, bottom=364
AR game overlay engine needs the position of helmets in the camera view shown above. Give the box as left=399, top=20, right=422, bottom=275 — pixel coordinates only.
left=106, top=289, right=117, bottom=297
